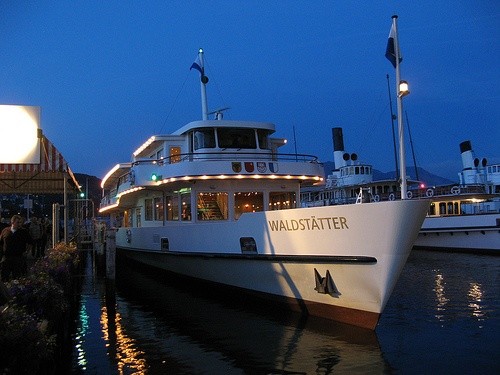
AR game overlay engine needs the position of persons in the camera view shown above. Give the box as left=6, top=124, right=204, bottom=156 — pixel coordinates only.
left=0, top=215, right=32, bottom=282
left=0, top=214, right=52, bottom=258
left=179, top=203, right=191, bottom=221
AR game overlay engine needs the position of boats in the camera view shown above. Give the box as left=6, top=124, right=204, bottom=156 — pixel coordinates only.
left=411, top=185, right=500, bottom=252
left=96, top=17, right=436, bottom=331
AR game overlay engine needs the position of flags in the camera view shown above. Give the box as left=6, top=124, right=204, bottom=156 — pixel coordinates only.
left=189, top=54, right=207, bottom=85
left=385, top=22, right=403, bottom=69
